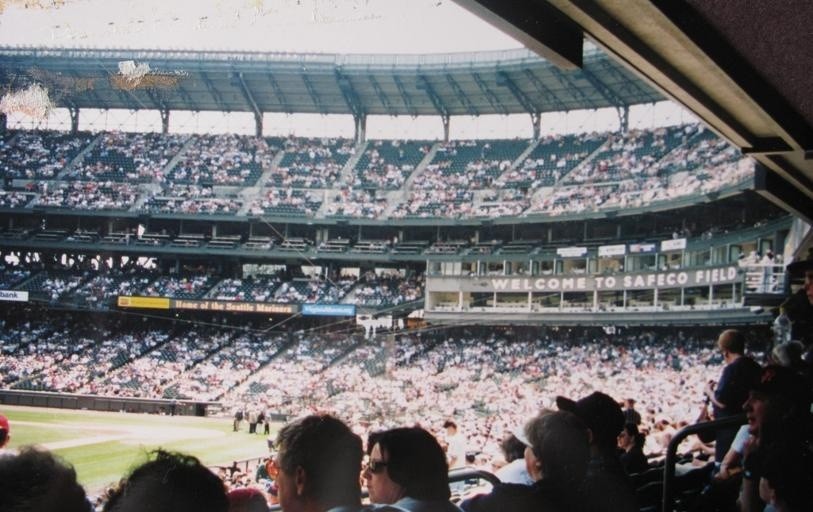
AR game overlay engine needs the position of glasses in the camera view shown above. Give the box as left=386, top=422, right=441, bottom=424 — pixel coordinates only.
left=369, top=460, right=386, bottom=471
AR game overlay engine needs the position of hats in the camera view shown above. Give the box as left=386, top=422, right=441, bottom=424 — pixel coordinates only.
left=555, top=393, right=623, bottom=452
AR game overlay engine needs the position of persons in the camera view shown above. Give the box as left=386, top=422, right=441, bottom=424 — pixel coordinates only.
left=2, top=258, right=813, bottom=512
left=1, top=126, right=748, bottom=256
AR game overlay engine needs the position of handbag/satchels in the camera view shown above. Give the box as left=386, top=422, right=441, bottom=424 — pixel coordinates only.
left=695, top=408, right=716, bottom=443
